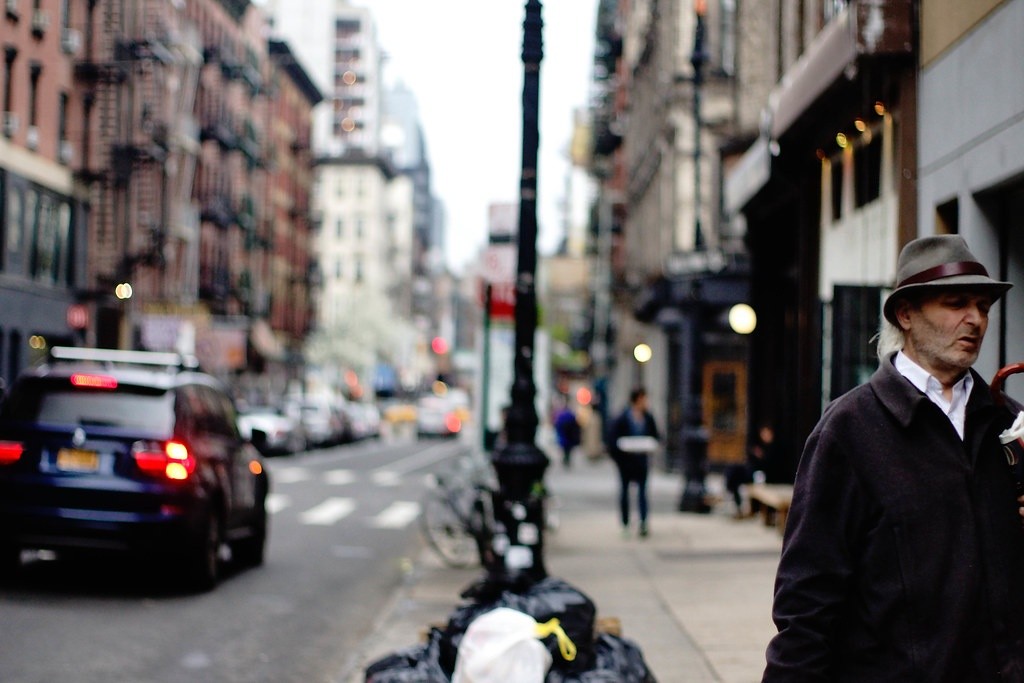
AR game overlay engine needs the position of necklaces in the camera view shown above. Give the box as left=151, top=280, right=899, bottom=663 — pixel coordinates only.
left=758, top=235, right=1024, bottom=683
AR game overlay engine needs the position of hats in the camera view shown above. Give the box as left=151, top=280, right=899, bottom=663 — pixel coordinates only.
left=884, top=234, right=1014, bottom=330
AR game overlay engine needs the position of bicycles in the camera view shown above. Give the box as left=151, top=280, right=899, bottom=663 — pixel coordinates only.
left=418, top=452, right=507, bottom=569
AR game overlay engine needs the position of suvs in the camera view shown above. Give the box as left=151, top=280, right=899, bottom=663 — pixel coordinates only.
left=0, top=347, right=272, bottom=593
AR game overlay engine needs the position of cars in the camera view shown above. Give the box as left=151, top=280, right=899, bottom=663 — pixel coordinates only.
left=236, top=388, right=467, bottom=454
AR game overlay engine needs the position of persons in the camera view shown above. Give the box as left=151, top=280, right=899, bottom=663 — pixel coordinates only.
left=729, top=424, right=786, bottom=515
left=606, top=387, right=659, bottom=533
left=554, top=403, right=581, bottom=467
